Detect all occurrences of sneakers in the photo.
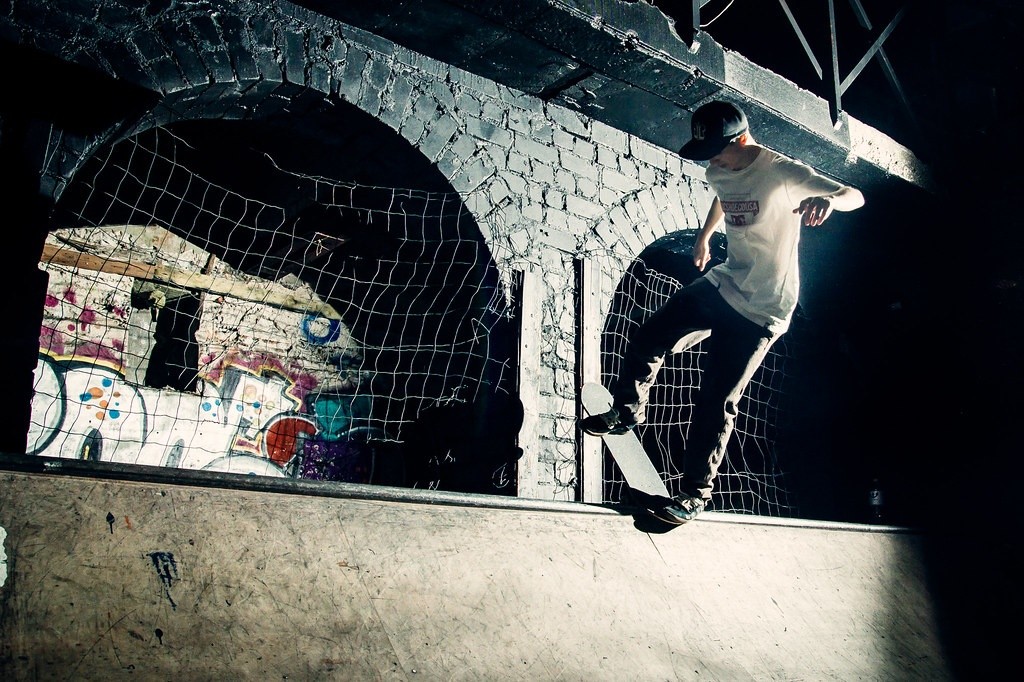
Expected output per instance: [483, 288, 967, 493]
[582, 407, 631, 435]
[663, 491, 705, 522]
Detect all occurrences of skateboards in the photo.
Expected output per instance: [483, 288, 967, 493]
[581, 383, 683, 525]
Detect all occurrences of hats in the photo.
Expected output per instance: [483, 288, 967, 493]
[678, 99, 750, 161]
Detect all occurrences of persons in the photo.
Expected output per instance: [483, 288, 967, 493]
[579, 98, 866, 527]
